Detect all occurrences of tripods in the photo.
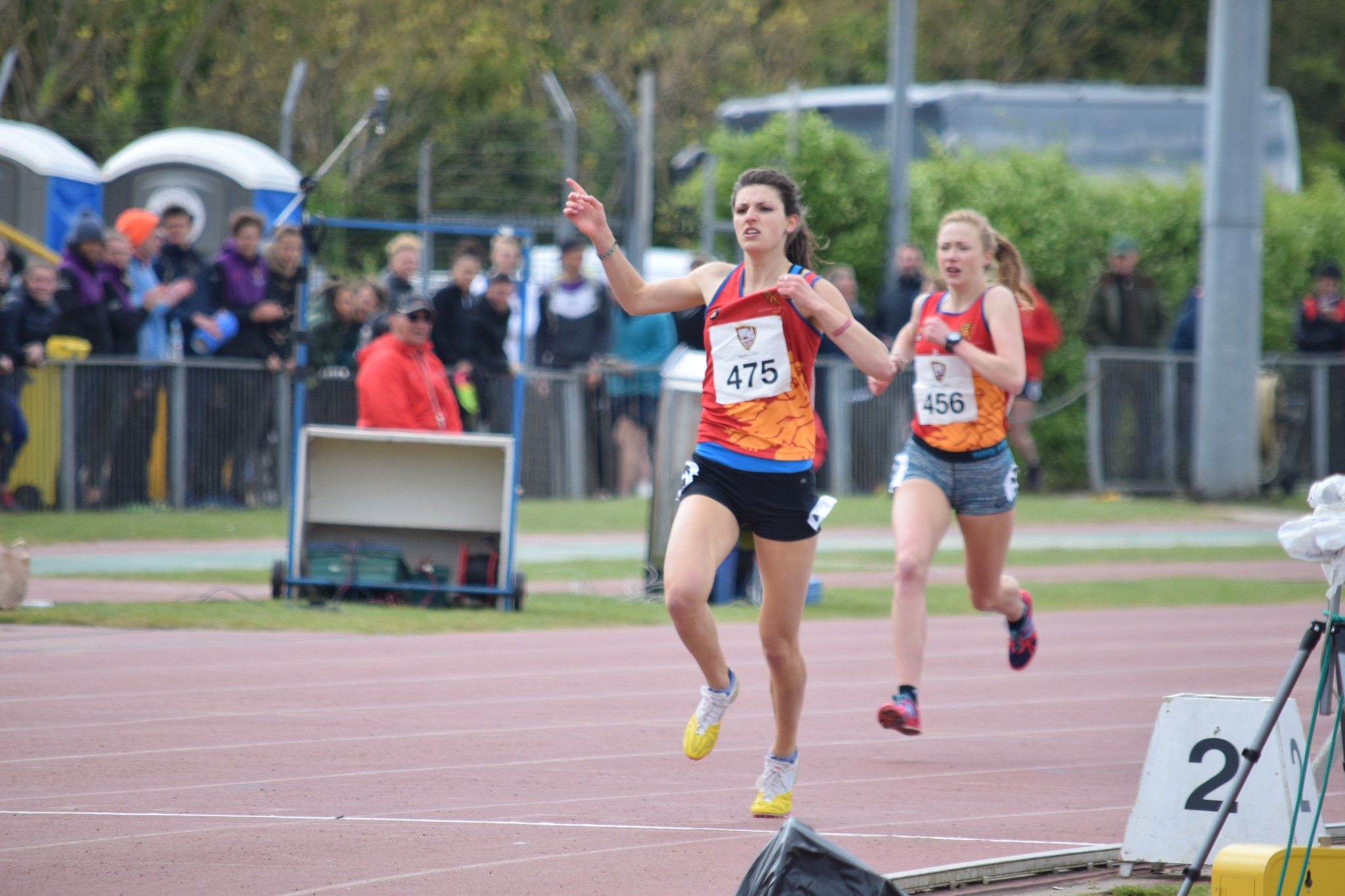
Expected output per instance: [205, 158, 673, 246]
[1178, 588, 1345, 896]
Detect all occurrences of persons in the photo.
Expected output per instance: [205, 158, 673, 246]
[869, 209, 1039, 738]
[1, 204, 1345, 522]
[564, 167, 895, 817]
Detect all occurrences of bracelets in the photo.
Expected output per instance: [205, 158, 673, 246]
[891, 355, 900, 374]
[897, 352, 906, 371]
[598, 240, 618, 260]
[829, 318, 853, 337]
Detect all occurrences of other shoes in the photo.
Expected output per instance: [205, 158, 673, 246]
[1026, 459, 1041, 489]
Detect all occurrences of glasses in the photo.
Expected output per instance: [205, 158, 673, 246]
[406, 311, 433, 322]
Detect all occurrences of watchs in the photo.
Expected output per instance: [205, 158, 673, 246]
[944, 332, 962, 352]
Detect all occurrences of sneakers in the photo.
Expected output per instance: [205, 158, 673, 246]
[681, 664, 739, 760]
[1005, 589, 1038, 671]
[878, 693, 922, 737]
[751, 750, 799, 817]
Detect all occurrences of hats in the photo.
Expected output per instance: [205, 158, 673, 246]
[1109, 238, 1137, 258]
[115, 208, 160, 246]
[73, 211, 106, 248]
[395, 292, 435, 315]
[1304, 259, 1341, 279]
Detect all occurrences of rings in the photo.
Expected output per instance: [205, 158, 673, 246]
[784, 274, 788, 281]
